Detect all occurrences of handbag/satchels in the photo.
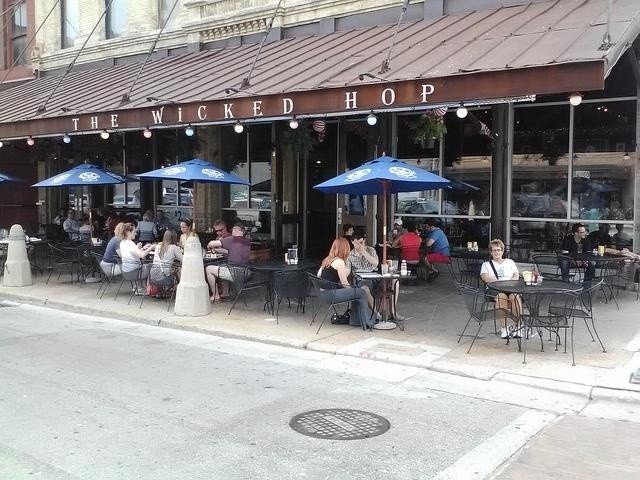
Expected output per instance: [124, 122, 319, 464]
[331, 308, 351, 324]
[164, 273, 179, 291]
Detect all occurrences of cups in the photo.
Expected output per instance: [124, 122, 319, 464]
[381, 264, 388, 275]
[536, 276, 543, 286]
[205, 254, 210, 258]
[598, 246, 605, 256]
[578, 244, 583, 253]
[467, 242, 479, 252]
[392, 260, 398, 269]
[523, 271, 532, 286]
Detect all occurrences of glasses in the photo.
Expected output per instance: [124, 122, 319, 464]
[492, 249, 501, 252]
[215, 229, 223, 232]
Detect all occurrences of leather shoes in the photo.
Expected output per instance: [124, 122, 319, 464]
[393, 313, 404, 320]
[434, 269, 440, 279]
[427, 273, 435, 283]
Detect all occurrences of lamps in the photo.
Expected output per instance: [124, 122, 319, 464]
[101, 128, 109, 140]
[144, 125, 152, 139]
[185, 123, 194, 137]
[568, 92, 584, 106]
[63, 131, 71, 143]
[26, 135, 34, 146]
[234, 119, 243, 134]
[367, 108, 377, 126]
[289, 115, 298, 130]
[457, 100, 468, 119]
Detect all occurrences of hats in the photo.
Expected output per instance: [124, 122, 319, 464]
[393, 219, 403, 226]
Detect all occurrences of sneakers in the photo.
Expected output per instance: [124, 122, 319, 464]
[221, 296, 230, 301]
[500, 326, 510, 338]
[513, 326, 523, 338]
[134, 288, 146, 295]
[211, 298, 221, 303]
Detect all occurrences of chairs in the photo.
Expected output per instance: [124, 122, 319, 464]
[0, 222, 318, 325]
[306, 269, 366, 335]
[375, 239, 640, 366]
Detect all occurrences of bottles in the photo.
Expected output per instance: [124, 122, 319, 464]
[533, 271, 540, 285]
[393, 230, 397, 241]
[401, 260, 407, 277]
[593, 249, 598, 256]
[93, 238, 103, 247]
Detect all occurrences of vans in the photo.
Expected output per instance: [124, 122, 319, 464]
[173, 187, 193, 207]
[112, 195, 138, 206]
[132, 187, 177, 205]
[454, 192, 564, 230]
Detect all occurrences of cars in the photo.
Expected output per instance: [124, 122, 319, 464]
[398, 197, 428, 213]
[397, 201, 470, 247]
[228, 192, 272, 209]
[558, 200, 580, 219]
[68, 193, 89, 208]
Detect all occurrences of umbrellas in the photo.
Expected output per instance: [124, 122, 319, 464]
[537, 175, 619, 219]
[313, 152, 453, 323]
[135, 157, 252, 238]
[252, 178, 271, 194]
[0, 172, 24, 183]
[419, 176, 481, 226]
[30, 160, 140, 278]
[178, 180, 193, 189]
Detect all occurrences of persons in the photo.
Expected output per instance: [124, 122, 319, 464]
[390, 219, 450, 282]
[587, 223, 632, 252]
[317, 238, 369, 327]
[480, 237, 522, 339]
[343, 223, 354, 250]
[150, 229, 183, 291]
[622, 247, 640, 291]
[179, 217, 199, 245]
[206, 220, 250, 304]
[101, 222, 156, 296]
[346, 231, 405, 321]
[135, 210, 168, 242]
[558, 222, 595, 292]
[55, 208, 121, 244]
